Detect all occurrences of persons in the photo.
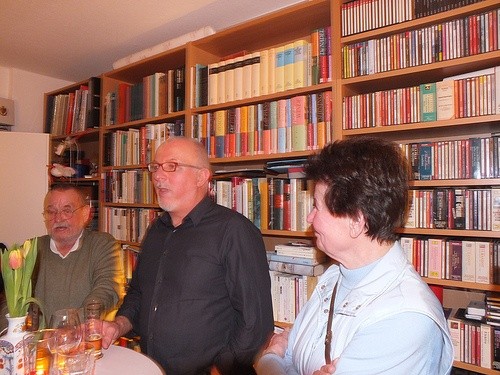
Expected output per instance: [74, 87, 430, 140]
[18, 183, 124, 329]
[254, 136, 454, 375]
[69, 136, 275, 375]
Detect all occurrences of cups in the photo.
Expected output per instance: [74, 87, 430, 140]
[53, 343, 95, 374]
[84, 299, 104, 360]
[48, 308, 83, 354]
[25, 305, 33, 331]
[24, 328, 58, 375]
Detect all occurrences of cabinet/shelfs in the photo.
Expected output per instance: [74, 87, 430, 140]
[41, 1, 500, 375]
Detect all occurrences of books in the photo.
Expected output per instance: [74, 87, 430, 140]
[209, 157, 317, 232]
[405, 185, 500, 231]
[117, 332, 143, 353]
[102, 66, 184, 126]
[189, 25, 333, 110]
[99, 168, 160, 205]
[396, 235, 500, 284]
[342, 64, 500, 181]
[103, 120, 183, 166]
[190, 91, 333, 159]
[119, 245, 141, 285]
[428, 285, 500, 370]
[340, 0, 500, 79]
[45, 76, 99, 136]
[262, 240, 342, 336]
[102, 207, 166, 243]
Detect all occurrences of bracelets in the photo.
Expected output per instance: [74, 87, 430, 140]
[257, 351, 276, 356]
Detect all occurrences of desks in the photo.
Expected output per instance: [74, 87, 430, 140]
[0, 332, 167, 375]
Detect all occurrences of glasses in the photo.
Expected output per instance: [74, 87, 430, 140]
[146, 162, 203, 172]
[42, 204, 88, 220]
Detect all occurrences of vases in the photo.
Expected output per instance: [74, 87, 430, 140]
[4, 313, 28, 345]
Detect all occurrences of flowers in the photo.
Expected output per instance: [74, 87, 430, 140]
[0, 236, 45, 351]
